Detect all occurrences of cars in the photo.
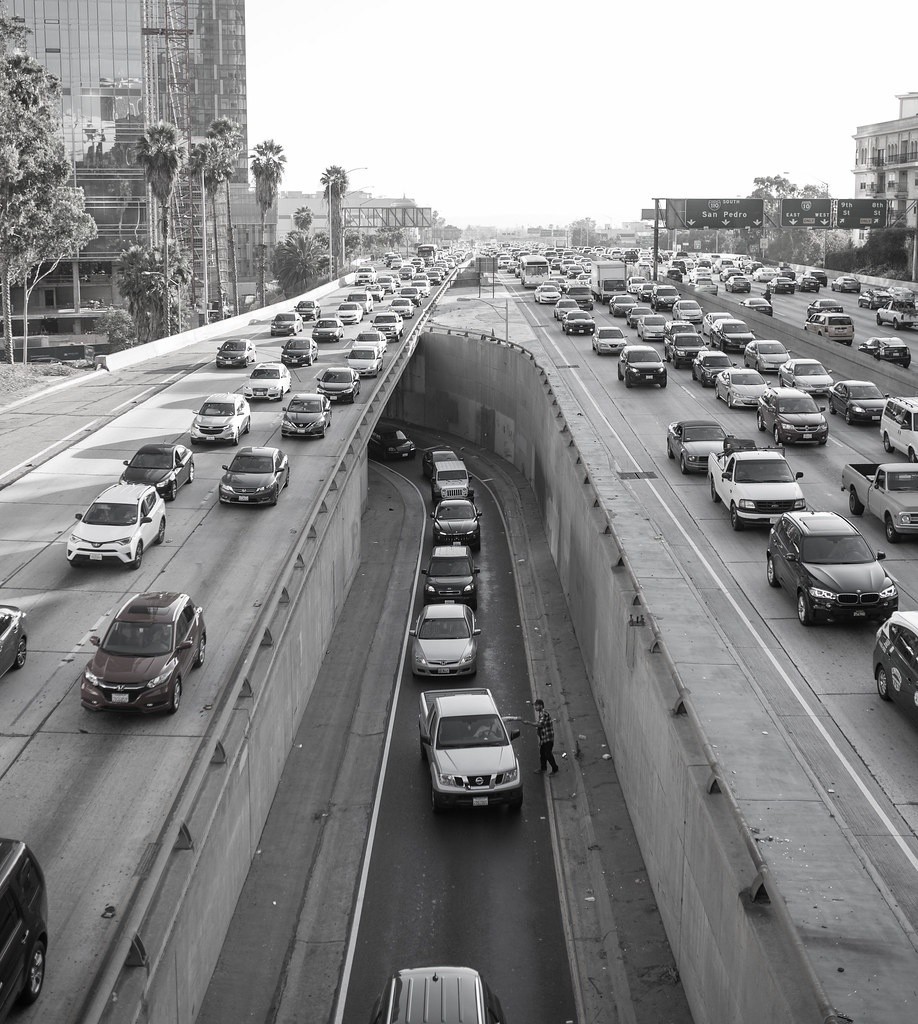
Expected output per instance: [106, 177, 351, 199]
[316, 368, 361, 405]
[280, 394, 332, 439]
[1, 837, 50, 1024]
[371, 965, 507, 1024]
[218, 446, 289, 507]
[190, 394, 251, 446]
[79, 589, 207, 720]
[408, 604, 481, 680]
[119, 444, 195, 503]
[484, 237, 918, 723]
[367, 424, 482, 613]
[243, 362, 292, 402]
[214, 246, 466, 379]
[0, 604, 29, 677]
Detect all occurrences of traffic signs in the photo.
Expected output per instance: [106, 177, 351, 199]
[684, 198, 889, 229]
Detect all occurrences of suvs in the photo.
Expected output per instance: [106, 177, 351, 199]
[65, 483, 166, 571]
[757, 387, 829, 446]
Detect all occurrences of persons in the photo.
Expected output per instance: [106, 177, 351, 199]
[523, 699, 559, 775]
[161, 455, 172, 468]
[149, 627, 171, 651]
[841, 538, 869, 559]
[259, 462, 272, 470]
[800, 400, 816, 413]
[473, 716, 522, 738]
[125, 508, 144, 520]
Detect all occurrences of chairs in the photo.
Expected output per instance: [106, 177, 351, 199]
[119, 625, 140, 639]
[141, 455, 156, 466]
[836, 538, 867, 558]
[148, 623, 168, 641]
[447, 721, 466, 740]
[470, 717, 493, 737]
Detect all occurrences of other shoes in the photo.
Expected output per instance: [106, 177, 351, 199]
[535, 768, 545, 773]
[549, 772, 555, 776]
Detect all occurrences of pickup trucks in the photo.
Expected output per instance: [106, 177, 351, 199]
[839, 461, 918, 545]
[706, 447, 805, 531]
[418, 687, 524, 816]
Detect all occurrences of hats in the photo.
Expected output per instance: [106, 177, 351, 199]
[534, 699, 544, 706]
